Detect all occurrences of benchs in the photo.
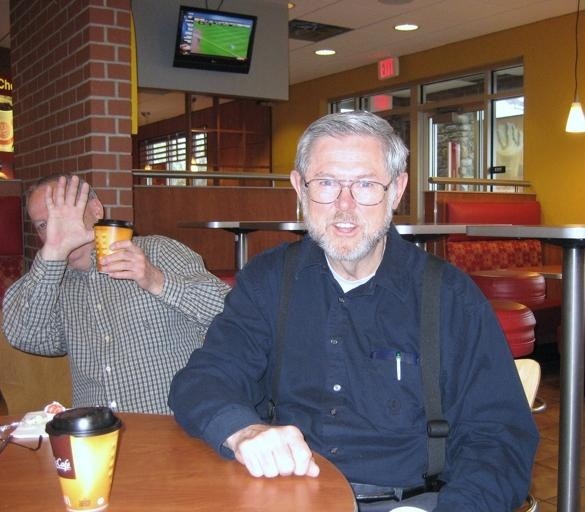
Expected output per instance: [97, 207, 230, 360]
[424, 192, 561, 345]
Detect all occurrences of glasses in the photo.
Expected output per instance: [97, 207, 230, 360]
[304, 179, 392, 206]
[0, 435, 42, 454]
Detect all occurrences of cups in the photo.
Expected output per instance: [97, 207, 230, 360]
[45, 405, 122, 512]
[92, 219, 134, 276]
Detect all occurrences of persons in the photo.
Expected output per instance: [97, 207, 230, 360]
[1, 171, 231, 416]
[163, 108, 537, 512]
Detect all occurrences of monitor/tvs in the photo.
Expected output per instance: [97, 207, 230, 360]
[173, 5, 257, 74]
